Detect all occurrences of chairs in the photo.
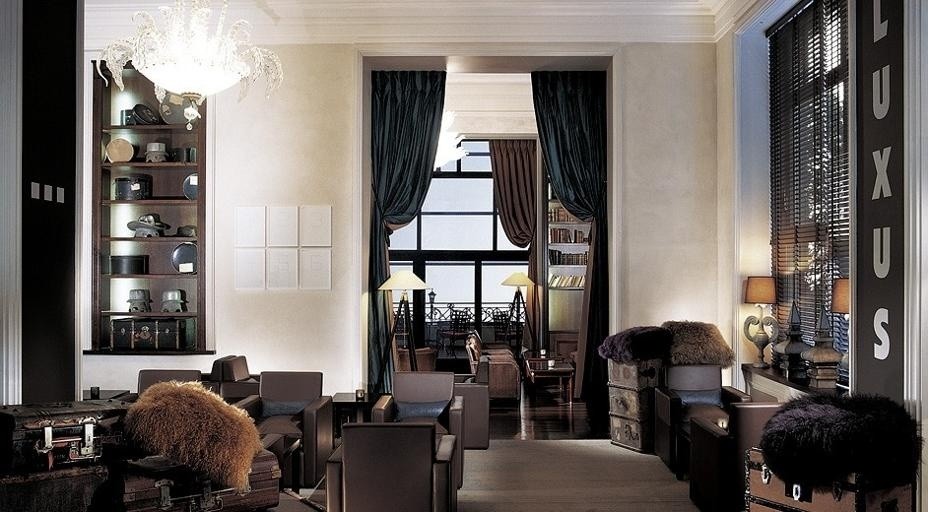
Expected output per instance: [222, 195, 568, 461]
[118, 370, 200, 404]
[200, 354, 236, 395]
[652, 362, 787, 512]
[325, 421, 457, 511]
[220, 356, 260, 404]
[465, 330, 519, 412]
[397, 348, 491, 450]
[435, 304, 523, 357]
[231, 371, 333, 492]
[371, 372, 464, 490]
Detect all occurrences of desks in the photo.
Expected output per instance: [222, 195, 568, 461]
[83, 390, 130, 400]
[331, 392, 378, 439]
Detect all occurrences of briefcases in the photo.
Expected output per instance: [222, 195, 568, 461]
[117, 448, 281, 511]
[744, 446, 917, 512]
[1, 399, 135, 470]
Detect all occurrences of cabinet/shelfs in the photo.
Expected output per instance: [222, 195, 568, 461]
[606, 357, 661, 454]
[91, 58, 208, 357]
[541, 175, 593, 358]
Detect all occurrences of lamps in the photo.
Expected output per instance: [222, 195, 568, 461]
[376, 270, 433, 395]
[829, 277, 850, 398]
[500, 272, 535, 360]
[92, 0, 286, 122]
[743, 274, 780, 368]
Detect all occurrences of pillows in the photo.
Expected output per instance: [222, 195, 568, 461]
[757, 390, 923, 495]
[597, 319, 737, 370]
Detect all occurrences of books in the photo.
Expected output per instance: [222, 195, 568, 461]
[548, 180, 594, 288]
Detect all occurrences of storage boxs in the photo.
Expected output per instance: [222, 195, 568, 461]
[101, 441, 281, 511]
